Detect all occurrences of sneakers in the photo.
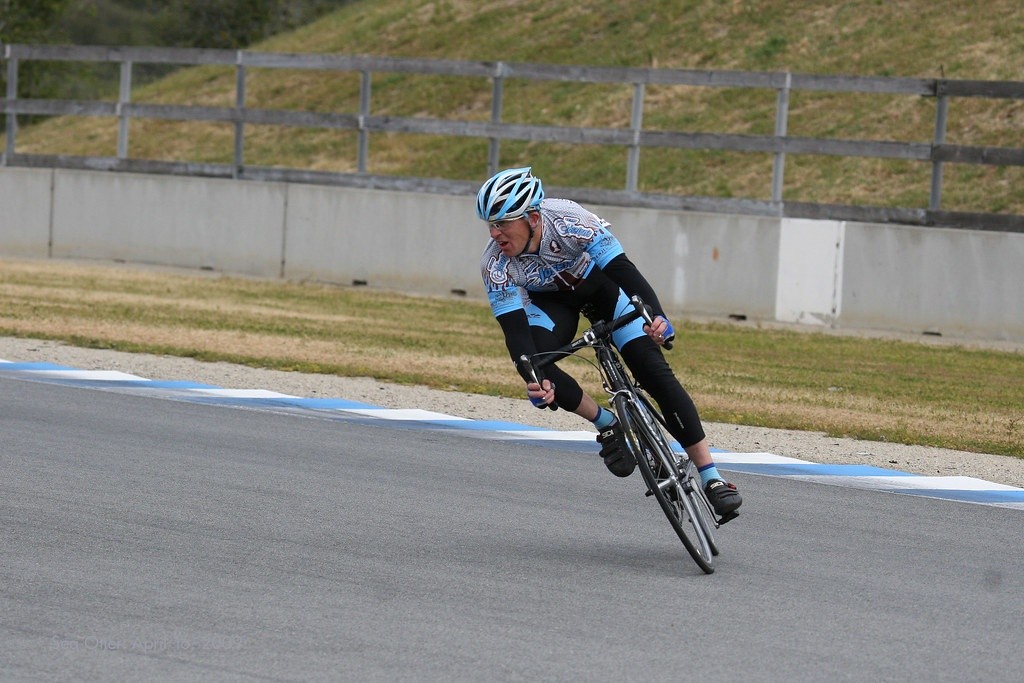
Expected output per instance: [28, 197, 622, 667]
[597, 407, 636, 477]
[705, 480, 742, 514]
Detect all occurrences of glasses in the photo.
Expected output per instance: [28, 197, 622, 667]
[488, 220, 513, 231]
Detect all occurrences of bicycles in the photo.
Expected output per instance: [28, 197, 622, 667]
[516, 292, 740, 574]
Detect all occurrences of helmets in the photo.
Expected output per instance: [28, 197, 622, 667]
[475, 167, 544, 221]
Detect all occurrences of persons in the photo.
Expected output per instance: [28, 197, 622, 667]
[476, 167, 742, 516]
